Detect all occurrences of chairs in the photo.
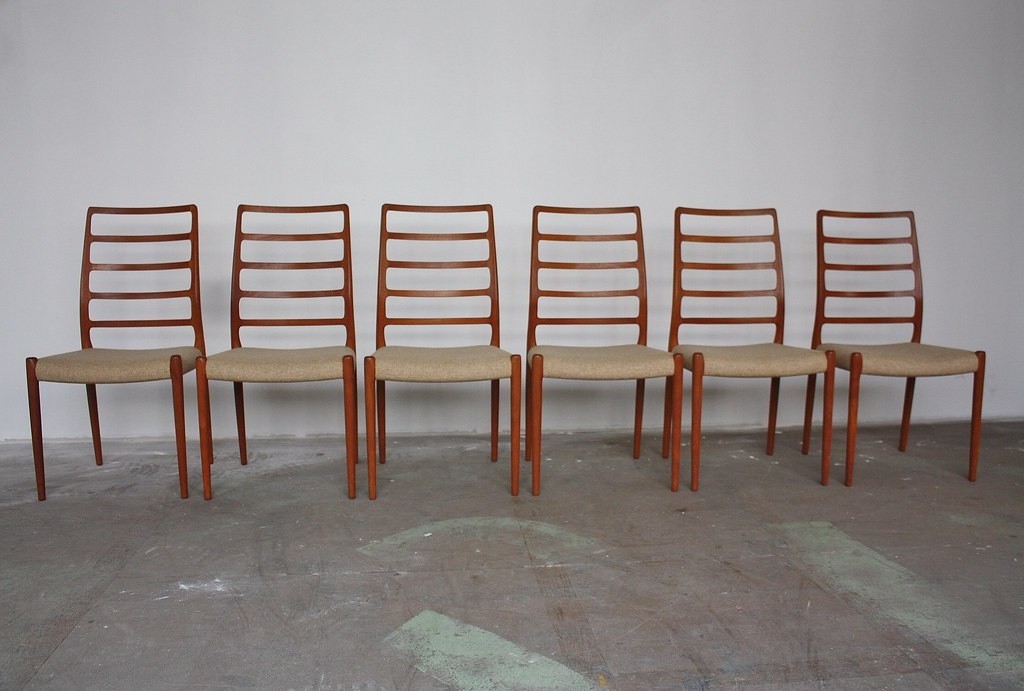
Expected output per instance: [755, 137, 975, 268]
[195, 203, 358, 500]
[662, 207, 836, 492]
[25, 204, 213, 501]
[525, 206, 685, 496]
[801, 209, 987, 487]
[363, 203, 521, 500]
